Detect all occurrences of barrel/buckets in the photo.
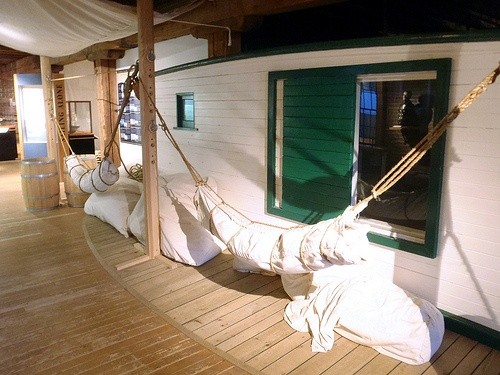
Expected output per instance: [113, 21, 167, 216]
[62, 153, 97, 209]
[21, 158, 59, 211]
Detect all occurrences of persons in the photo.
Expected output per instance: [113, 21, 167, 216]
[398, 90, 415, 126]
